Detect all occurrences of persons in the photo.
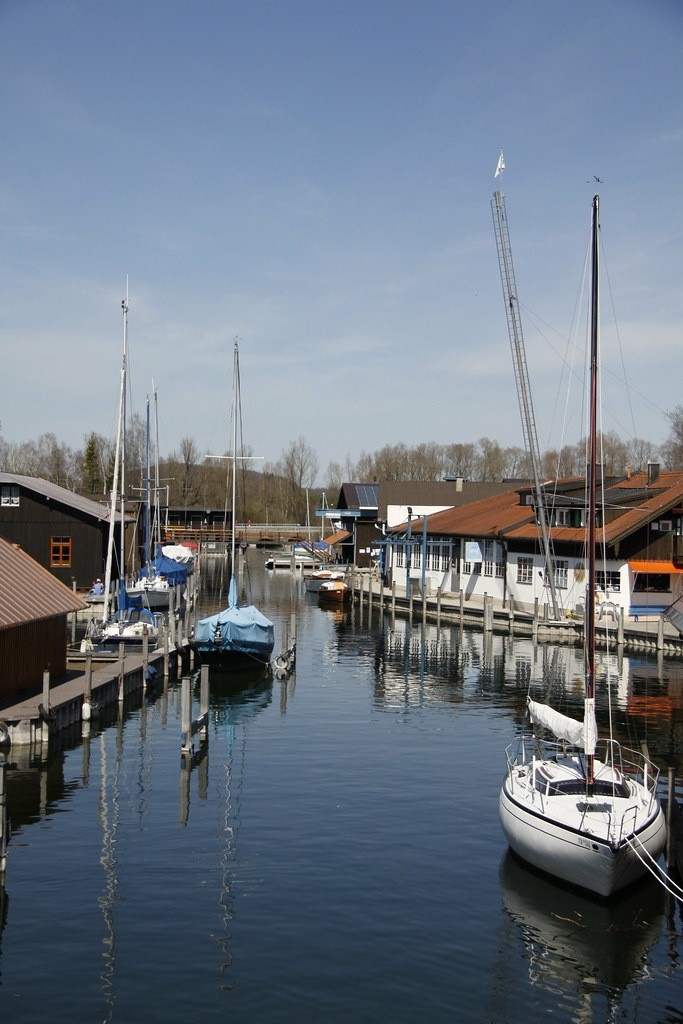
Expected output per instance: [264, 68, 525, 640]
[92, 578, 104, 595]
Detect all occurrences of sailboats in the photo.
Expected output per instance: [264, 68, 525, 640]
[499, 166, 672, 901]
[265, 489, 364, 601]
[190, 335, 275, 671]
[94, 272, 197, 640]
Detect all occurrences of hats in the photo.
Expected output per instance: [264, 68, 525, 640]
[95, 579, 102, 582]
